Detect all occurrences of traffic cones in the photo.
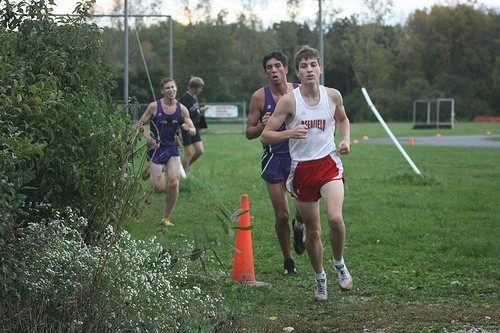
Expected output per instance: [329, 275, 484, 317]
[409, 137, 414, 145]
[230, 193, 257, 284]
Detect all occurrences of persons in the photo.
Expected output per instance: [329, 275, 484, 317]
[180, 77, 208, 170]
[259, 46, 354, 303]
[245, 50, 307, 276]
[136, 77, 197, 227]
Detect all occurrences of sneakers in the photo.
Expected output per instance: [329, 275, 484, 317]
[314, 273, 328, 301]
[333, 262, 352, 290]
[283, 257, 296, 275]
[142, 166, 151, 180]
[159, 218, 175, 226]
[292, 219, 306, 255]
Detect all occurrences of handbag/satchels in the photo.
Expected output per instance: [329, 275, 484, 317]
[200, 104, 208, 129]
